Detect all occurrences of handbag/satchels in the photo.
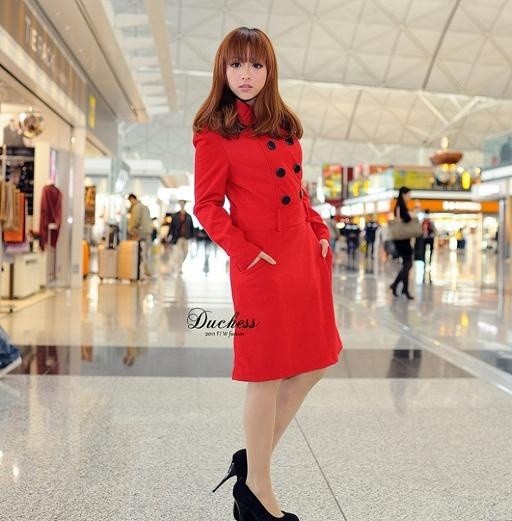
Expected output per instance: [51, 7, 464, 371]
[382, 204, 423, 241]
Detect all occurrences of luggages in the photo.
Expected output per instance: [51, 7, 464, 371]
[116, 239, 146, 281]
[97, 244, 117, 280]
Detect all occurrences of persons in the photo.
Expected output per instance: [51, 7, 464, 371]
[190, 25, 345, 521]
[0, 327, 22, 381]
[125, 194, 230, 279]
[385, 331, 422, 409]
[325, 187, 468, 300]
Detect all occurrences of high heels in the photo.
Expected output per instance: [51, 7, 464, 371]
[402, 288, 415, 299]
[232, 478, 300, 521]
[389, 283, 399, 296]
[213, 448, 247, 521]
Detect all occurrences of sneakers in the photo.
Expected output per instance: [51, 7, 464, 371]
[0, 347, 22, 379]
